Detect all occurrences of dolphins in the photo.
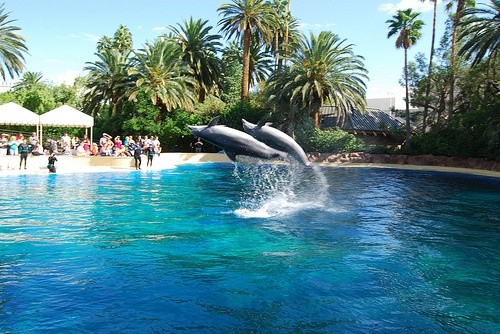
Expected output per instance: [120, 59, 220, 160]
[187, 115, 288, 163]
[241, 118, 312, 167]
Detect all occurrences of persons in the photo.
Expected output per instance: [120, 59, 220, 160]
[195, 141, 203, 153]
[47, 153, 57, 173]
[19, 139, 28, 170]
[0, 133, 164, 156]
[132, 140, 141, 169]
[145, 142, 155, 168]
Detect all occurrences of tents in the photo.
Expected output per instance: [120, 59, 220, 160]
[0, 102, 39, 139]
[40, 105, 94, 153]
